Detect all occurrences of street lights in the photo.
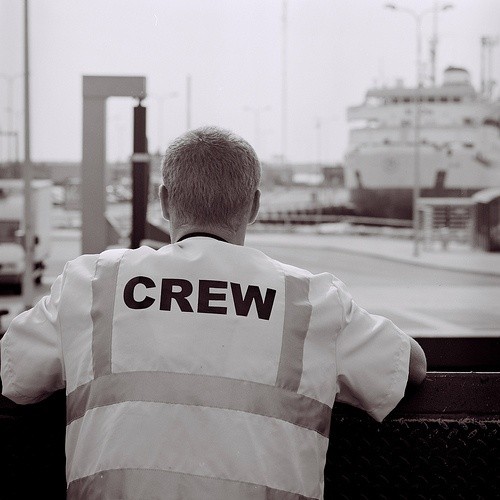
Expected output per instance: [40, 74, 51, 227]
[385, 1, 455, 257]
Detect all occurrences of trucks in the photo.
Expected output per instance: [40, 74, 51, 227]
[1, 178, 49, 294]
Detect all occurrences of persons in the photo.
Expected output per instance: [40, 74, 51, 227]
[0, 127, 426, 500]
